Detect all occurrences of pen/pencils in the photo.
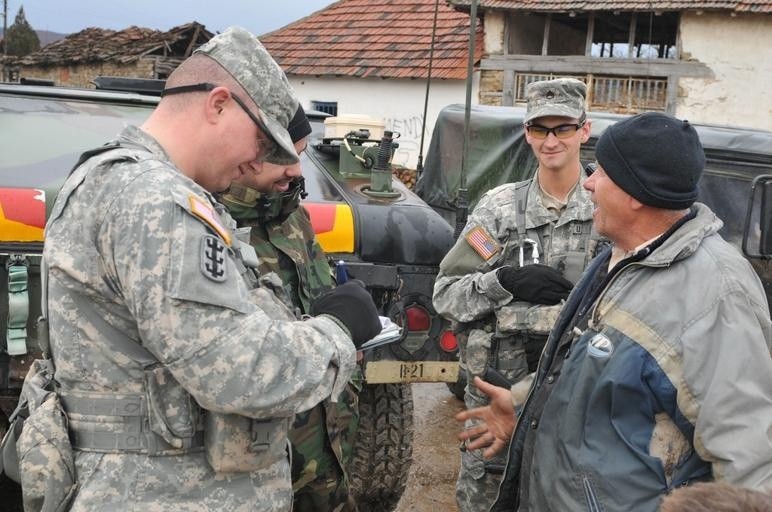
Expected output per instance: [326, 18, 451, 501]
[336, 259, 349, 283]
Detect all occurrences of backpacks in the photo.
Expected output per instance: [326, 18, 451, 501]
[14, 356, 78, 511]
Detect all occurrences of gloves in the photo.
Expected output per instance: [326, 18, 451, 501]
[525, 339, 546, 373]
[497, 263, 574, 305]
[309, 279, 382, 347]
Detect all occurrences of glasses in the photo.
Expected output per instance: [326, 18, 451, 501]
[204, 81, 278, 162]
[524, 119, 585, 140]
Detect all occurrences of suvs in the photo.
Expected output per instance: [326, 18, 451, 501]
[1, 2, 477, 512]
[410, 1, 771, 406]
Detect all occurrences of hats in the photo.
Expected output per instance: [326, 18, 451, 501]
[192, 25, 312, 165]
[524, 78, 588, 124]
[594, 112, 705, 210]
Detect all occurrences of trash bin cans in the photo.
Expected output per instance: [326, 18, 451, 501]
[323, 114, 385, 146]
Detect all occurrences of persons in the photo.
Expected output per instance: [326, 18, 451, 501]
[43, 24, 384, 510]
[449, 109, 771, 511]
[218, 98, 370, 511]
[654, 476, 770, 511]
[428, 76, 610, 510]
